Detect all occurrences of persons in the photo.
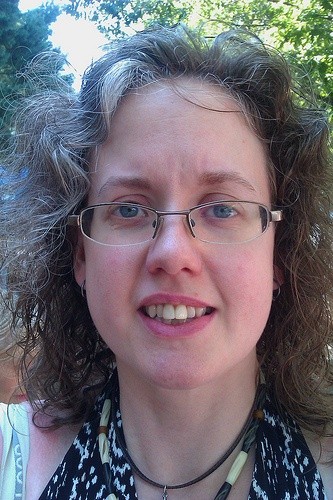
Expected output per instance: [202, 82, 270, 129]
[0, 21, 332, 500]
[0, 288, 46, 405]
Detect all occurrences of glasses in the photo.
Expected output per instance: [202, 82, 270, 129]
[76, 201, 282, 245]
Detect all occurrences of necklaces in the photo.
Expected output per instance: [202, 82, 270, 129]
[99, 366, 266, 500]
